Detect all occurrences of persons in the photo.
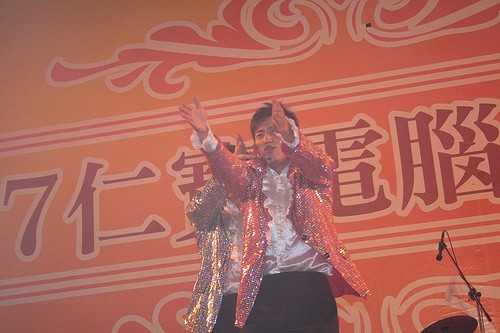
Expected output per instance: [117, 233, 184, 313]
[181, 134, 247, 333]
[179, 94, 372, 333]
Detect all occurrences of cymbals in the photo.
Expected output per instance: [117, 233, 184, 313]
[420, 315, 477, 333]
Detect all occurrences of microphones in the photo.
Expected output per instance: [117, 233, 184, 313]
[262, 157, 271, 160]
[436, 232, 445, 261]
